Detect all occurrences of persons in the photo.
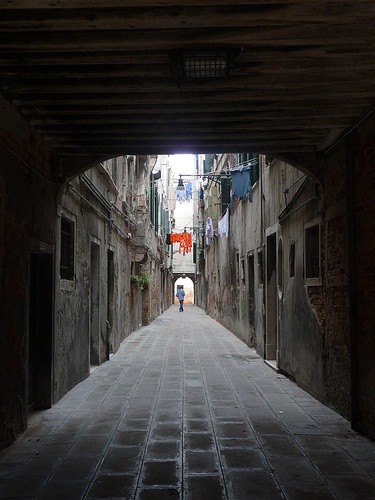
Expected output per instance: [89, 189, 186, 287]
[176, 285, 185, 312]
